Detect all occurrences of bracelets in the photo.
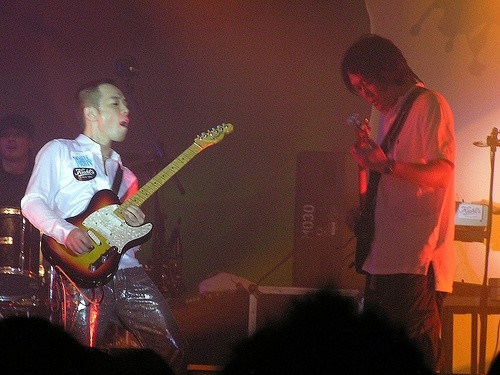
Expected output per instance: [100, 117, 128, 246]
[381, 158, 395, 174]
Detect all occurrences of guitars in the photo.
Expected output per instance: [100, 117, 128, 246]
[41, 122, 234, 289]
[347, 112, 380, 275]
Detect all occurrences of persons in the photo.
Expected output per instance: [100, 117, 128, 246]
[340, 35, 455, 374]
[0, 110, 37, 207]
[20, 78, 188, 374]
[218, 285, 439, 373]
[0, 313, 125, 374]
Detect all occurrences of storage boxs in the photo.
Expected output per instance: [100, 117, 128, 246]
[248, 288, 358, 339]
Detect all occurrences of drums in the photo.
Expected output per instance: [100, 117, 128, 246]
[0, 206, 41, 302]
[0, 240, 65, 330]
